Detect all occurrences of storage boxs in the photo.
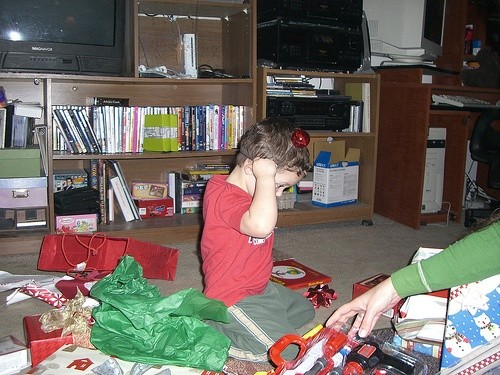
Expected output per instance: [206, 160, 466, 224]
[0, 334, 32, 375]
[13, 102, 42, 119]
[134, 196, 174, 219]
[52, 169, 88, 193]
[312, 148, 360, 208]
[132, 182, 168, 200]
[351, 273, 405, 317]
[54, 187, 102, 225]
[23, 315, 93, 368]
[0, 176, 48, 209]
[55, 213, 98, 234]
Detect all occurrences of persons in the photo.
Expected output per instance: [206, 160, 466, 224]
[199, 117, 317, 361]
[325, 221, 498, 340]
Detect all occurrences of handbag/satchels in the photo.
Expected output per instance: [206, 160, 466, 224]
[37, 235, 180, 281]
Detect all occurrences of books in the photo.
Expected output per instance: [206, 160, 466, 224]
[266, 74, 318, 97]
[0, 98, 251, 225]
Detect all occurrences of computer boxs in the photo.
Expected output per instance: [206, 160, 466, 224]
[422, 128, 447, 215]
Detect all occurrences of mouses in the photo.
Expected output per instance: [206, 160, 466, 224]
[496, 100, 500, 107]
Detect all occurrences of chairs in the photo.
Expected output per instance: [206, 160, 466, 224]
[465, 110, 500, 228]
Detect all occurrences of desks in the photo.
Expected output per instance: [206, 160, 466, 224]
[371, 64, 500, 231]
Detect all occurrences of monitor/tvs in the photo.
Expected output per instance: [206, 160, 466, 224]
[421, 0, 447, 61]
[0, 0, 135, 78]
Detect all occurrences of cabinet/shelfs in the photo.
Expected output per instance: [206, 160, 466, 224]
[0, 0, 381, 257]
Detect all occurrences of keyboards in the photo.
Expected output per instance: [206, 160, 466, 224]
[432, 93, 496, 108]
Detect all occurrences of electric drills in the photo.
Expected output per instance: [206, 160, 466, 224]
[343, 341, 414, 375]
